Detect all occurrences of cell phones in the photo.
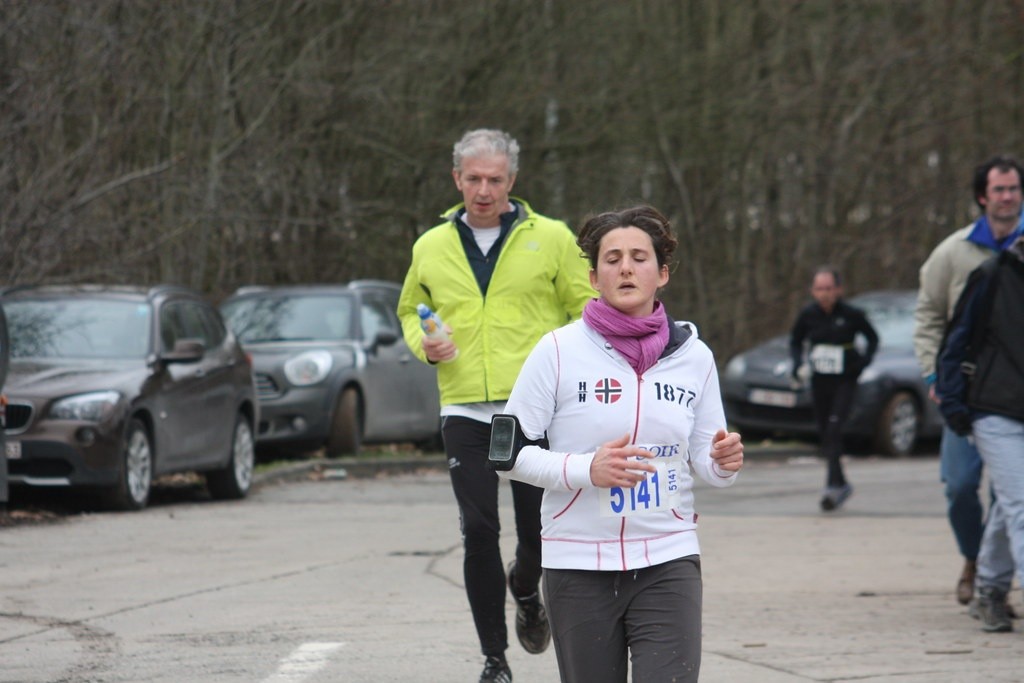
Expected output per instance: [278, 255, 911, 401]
[487, 414, 521, 471]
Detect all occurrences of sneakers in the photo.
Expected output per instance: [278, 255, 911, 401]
[967, 585, 1012, 632]
[479, 658, 513, 683]
[821, 482, 854, 512]
[507, 560, 552, 654]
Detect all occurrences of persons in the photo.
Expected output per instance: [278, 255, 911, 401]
[397, 129, 600, 683]
[487, 206, 744, 683]
[789, 269, 879, 511]
[913, 155, 1024, 632]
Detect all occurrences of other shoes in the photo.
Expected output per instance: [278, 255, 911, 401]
[1006, 602, 1023, 619]
[956, 563, 976, 604]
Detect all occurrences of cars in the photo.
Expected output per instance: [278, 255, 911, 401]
[719, 291, 945, 457]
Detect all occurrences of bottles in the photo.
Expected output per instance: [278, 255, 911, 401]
[417, 303, 459, 363]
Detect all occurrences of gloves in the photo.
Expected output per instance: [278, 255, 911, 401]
[792, 358, 806, 382]
[939, 399, 973, 437]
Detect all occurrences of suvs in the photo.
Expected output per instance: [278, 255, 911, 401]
[0, 282, 261, 509]
[212, 279, 445, 461]
